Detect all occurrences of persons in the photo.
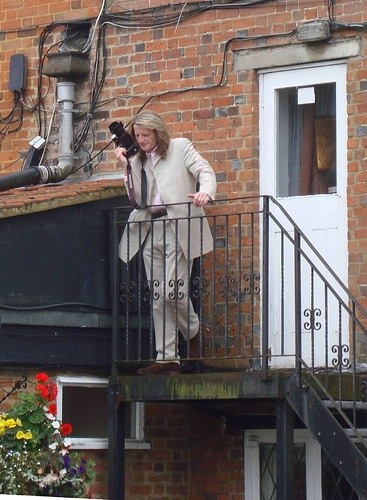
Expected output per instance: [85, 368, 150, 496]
[114, 110, 218, 375]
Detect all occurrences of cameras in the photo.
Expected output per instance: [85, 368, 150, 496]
[109, 121, 139, 159]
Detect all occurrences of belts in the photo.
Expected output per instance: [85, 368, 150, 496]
[151, 207, 168, 220]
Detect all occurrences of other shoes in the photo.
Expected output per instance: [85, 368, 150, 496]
[137, 361, 180, 375]
[179, 334, 203, 372]
